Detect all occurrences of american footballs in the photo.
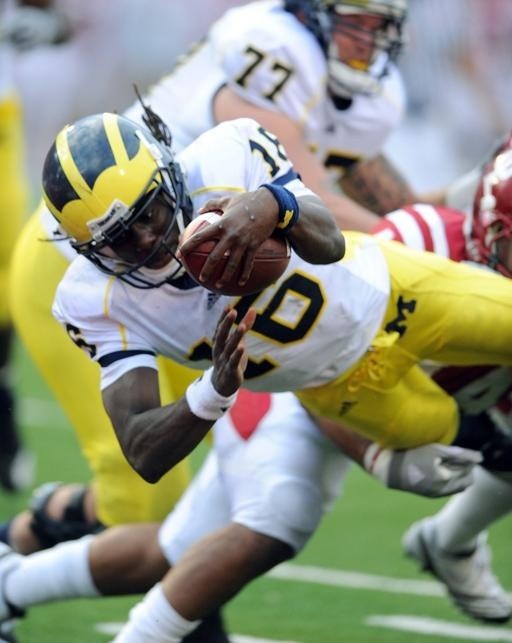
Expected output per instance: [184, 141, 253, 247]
[178, 211, 292, 294]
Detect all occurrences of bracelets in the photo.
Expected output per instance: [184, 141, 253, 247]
[185, 365, 239, 422]
[258, 183, 300, 235]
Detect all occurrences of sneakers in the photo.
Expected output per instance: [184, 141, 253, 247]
[401, 517, 512, 622]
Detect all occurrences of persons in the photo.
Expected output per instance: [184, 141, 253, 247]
[41, 112, 512, 483]
[0, 129, 512, 643]
[2, 1, 75, 497]
[1, 0, 446, 643]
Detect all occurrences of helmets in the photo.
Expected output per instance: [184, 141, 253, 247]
[42, 113, 193, 288]
[285, 0, 409, 99]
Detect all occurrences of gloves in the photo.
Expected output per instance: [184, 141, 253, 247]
[364, 443, 484, 498]
